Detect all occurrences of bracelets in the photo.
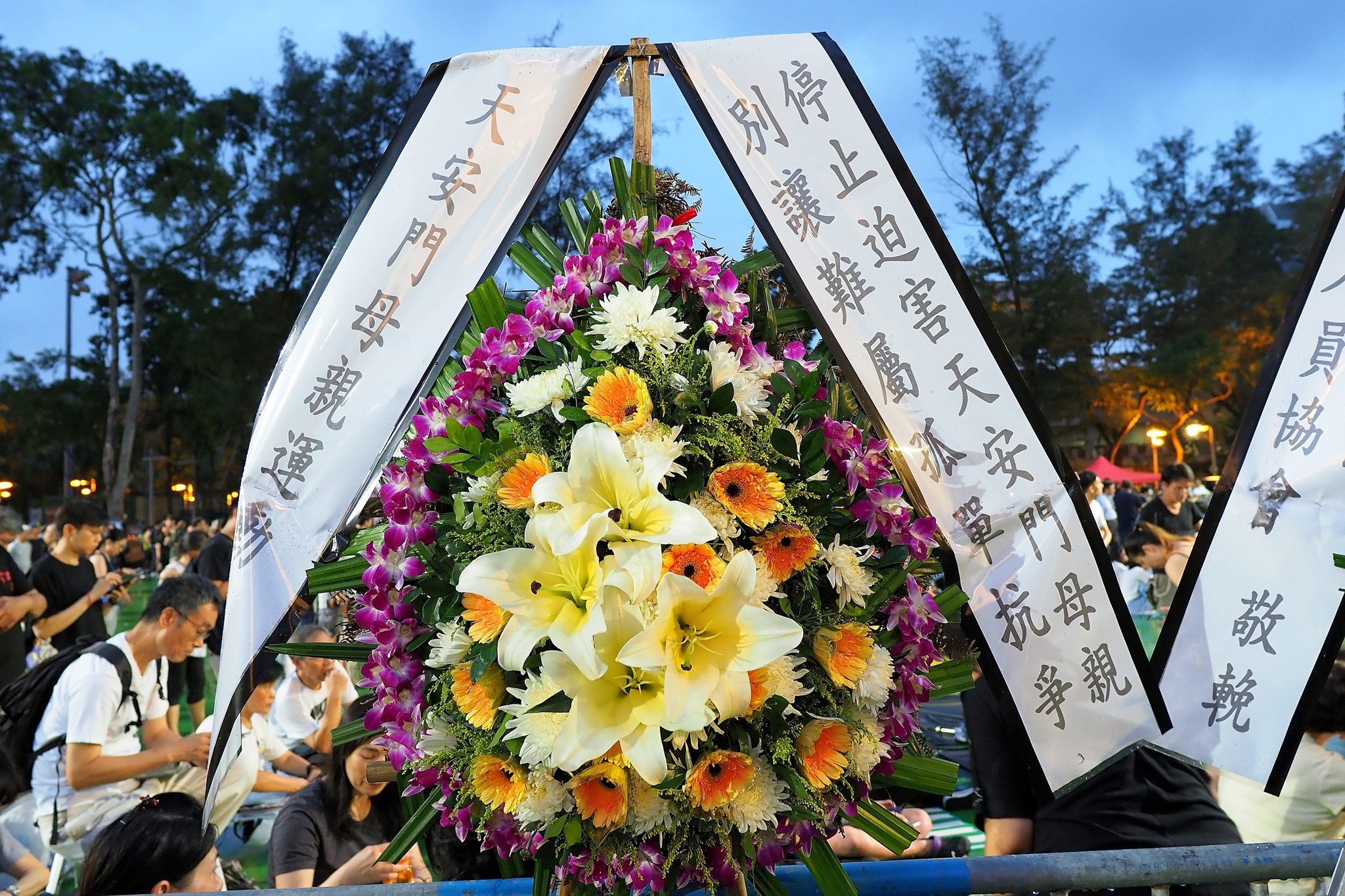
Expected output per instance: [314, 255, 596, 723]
[87, 592, 93, 605]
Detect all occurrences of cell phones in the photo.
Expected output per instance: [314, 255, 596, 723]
[106, 575, 134, 594]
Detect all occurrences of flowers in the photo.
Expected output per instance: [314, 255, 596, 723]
[265, 153, 984, 895]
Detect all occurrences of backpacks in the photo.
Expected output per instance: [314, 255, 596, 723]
[0, 632, 162, 805]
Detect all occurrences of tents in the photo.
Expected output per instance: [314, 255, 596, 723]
[1085, 455, 1164, 487]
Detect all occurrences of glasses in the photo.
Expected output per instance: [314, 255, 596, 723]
[174, 607, 211, 641]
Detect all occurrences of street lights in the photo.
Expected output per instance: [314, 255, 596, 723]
[1146, 427, 1169, 486]
[169, 483, 195, 522]
[61, 266, 92, 504]
[1186, 424, 1219, 490]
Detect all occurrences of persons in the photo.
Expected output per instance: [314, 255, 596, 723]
[1077, 461, 1219, 628]
[802, 800, 973, 859]
[1219, 665, 1345, 896]
[0, 496, 434, 896]
[937, 547, 1250, 896]
